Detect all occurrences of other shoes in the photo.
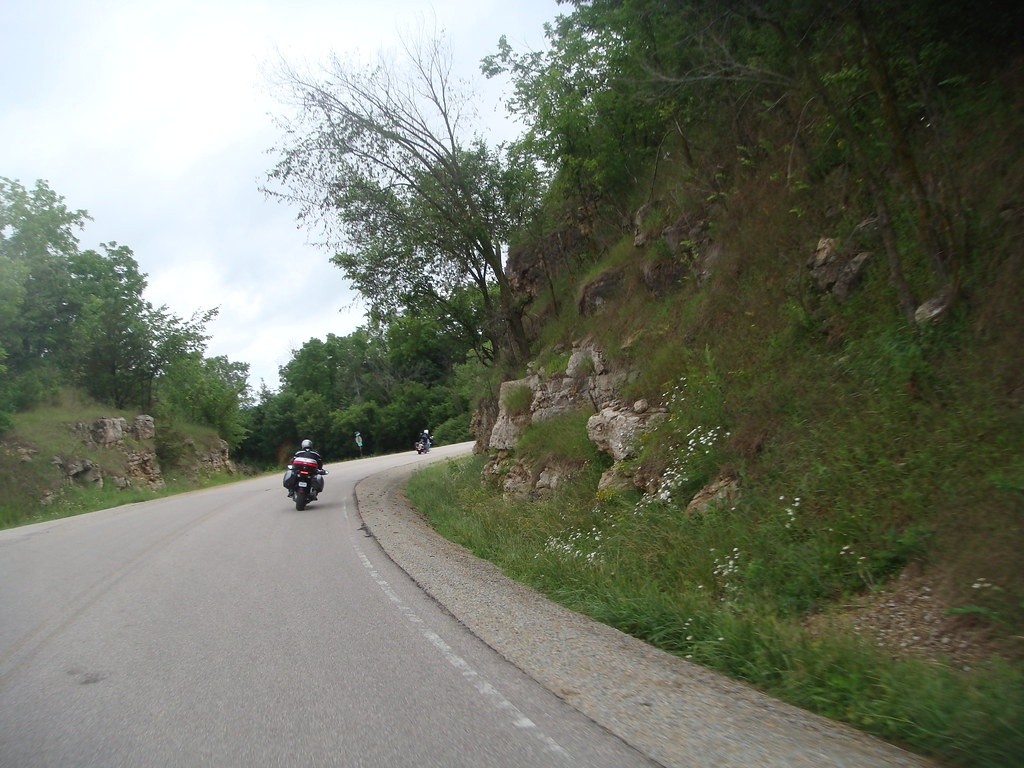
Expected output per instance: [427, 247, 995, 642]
[311, 495, 317, 500]
[287, 491, 294, 497]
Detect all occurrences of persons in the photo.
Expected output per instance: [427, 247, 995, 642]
[420, 430, 430, 452]
[285, 439, 323, 501]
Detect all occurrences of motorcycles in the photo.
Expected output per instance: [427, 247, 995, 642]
[415, 433, 434, 454]
[288, 457, 329, 511]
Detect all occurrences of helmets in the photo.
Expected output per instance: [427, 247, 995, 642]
[301, 439, 313, 449]
[424, 429, 429, 434]
